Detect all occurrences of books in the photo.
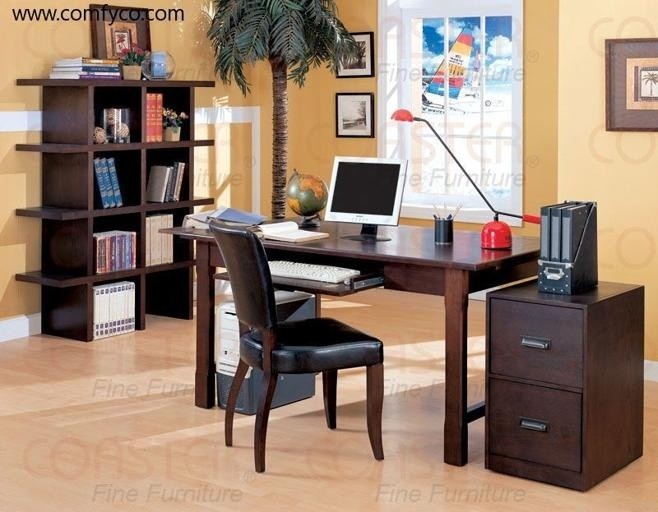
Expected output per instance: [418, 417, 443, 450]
[258, 221, 330, 243]
[49, 57, 121, 81]
[93, 92, 186, 275]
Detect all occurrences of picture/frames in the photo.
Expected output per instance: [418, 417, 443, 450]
[603, 37, 657, 131]
[334, 30, 375, 78]
[90, 2, 153, 61]
[333, 92, 374, 137]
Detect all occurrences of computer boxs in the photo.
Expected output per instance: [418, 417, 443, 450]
[215, 290, 316, 414]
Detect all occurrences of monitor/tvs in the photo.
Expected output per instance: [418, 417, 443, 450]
[325, 154, 408, 243]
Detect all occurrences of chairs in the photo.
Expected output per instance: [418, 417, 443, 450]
[205, 217, 388, 474]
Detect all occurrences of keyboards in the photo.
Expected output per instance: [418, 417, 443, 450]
[268, 259, 362, 284]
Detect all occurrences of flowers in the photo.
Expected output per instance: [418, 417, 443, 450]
[159, 105, 189, 133]
[116, 42, 147, 66]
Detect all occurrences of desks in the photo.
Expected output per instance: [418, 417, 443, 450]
[158, 211, 540, 468]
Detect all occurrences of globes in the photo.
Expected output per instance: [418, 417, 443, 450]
[286, 168, 328, 228]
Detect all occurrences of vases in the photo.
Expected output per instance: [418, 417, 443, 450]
[121, 65, 141, 80]
[162, 126, 180, 141]
[102, 106, 130, 144]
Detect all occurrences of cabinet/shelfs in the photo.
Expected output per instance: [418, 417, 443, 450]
[16, 75, 217, 342]
[485, 277, 643, 495]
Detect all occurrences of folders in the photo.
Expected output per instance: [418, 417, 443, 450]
[206, 206, 267, 226]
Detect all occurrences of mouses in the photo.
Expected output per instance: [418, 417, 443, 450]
[344, 278, 350, 286]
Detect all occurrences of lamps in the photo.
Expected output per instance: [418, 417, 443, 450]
[389, 109, 540, 250]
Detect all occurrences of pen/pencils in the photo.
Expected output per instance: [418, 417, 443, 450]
[432, 200, 462, 220]
